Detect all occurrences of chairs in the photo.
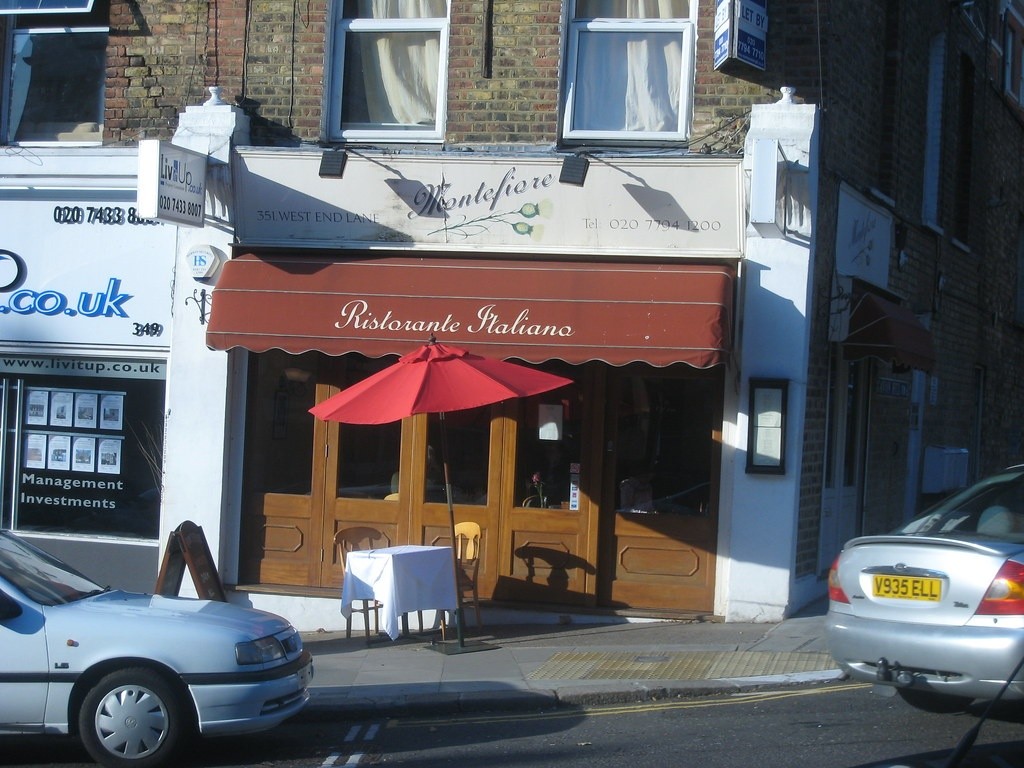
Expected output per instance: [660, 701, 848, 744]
[618, 479, 653, 512]
[332, 527, 392, 642]
[524, 495, 556, 509]
[431, 521, 482, 643]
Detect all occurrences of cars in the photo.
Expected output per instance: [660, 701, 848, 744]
[0, 530, 313, 768]
[824, 463, 1024, 714]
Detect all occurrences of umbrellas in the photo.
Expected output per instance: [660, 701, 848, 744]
[307, 332, 573, 645]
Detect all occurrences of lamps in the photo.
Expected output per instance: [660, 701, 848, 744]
[558, 151, 591, 186]
[317, 146, 348, 178]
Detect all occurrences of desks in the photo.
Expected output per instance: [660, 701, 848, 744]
[337, 544, 456, 643]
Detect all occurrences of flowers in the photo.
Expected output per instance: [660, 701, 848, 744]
[531, 472, 544, 506]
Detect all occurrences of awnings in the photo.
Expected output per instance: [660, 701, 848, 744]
[206, 254, 735, 369]
[841, 290, 935, 376]
